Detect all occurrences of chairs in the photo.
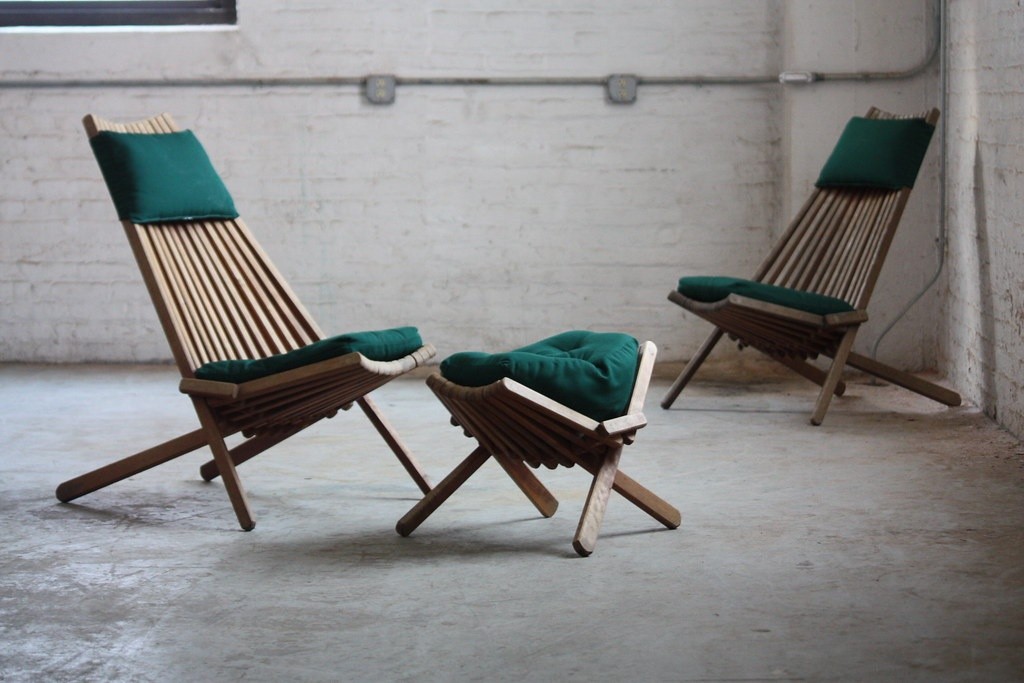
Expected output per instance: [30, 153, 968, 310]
[661, 106, 962, 428]
[55, 110, 438, 530]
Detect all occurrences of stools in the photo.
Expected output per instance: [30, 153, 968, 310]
[393, 329, 682, 556]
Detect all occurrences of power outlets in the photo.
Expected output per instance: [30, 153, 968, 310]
[608, 73, 637, 105]
[365, 73, 396, 105]
